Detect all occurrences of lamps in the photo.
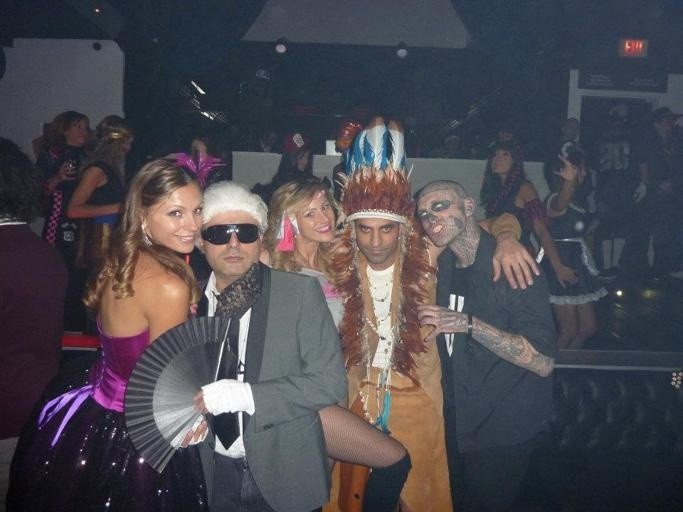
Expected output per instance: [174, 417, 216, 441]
[275, 36, 288, 55]
[396, 39, 408, 59]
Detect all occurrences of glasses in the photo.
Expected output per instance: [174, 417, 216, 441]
[200, 223, 260, 245]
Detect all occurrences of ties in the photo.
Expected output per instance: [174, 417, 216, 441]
[210, 305, 253, 450]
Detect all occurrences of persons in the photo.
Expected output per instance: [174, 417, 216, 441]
[479, 107, 682, 350]
[2, 110, 558, 510]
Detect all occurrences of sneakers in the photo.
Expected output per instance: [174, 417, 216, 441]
[603, 266, 617, 279]
